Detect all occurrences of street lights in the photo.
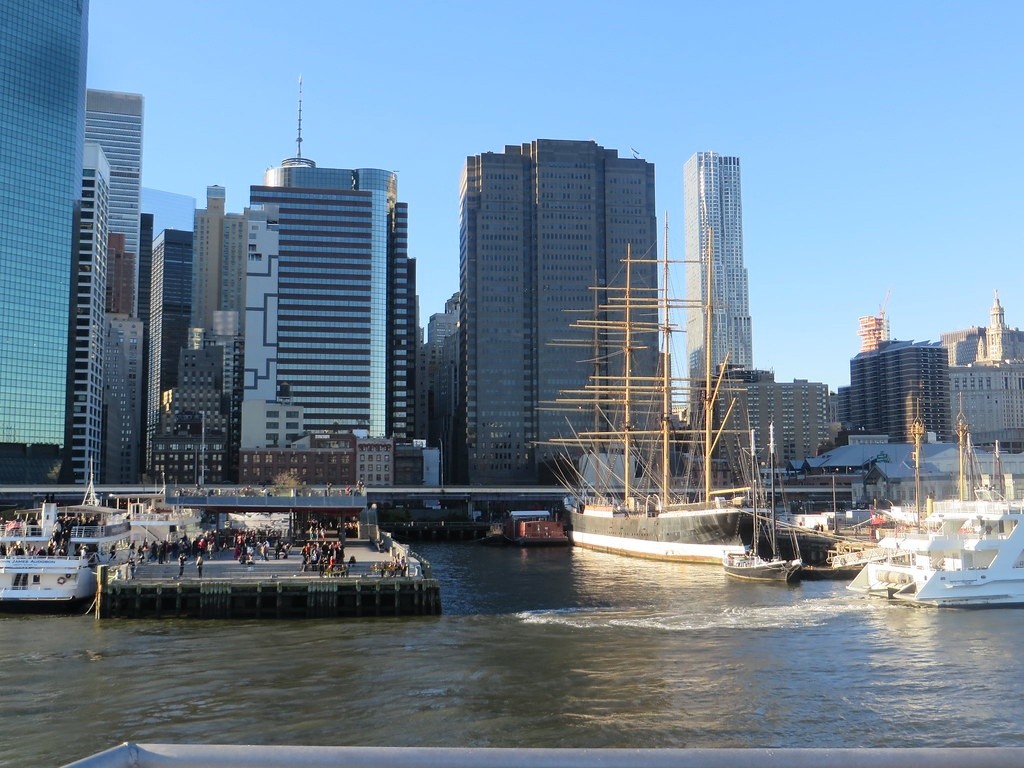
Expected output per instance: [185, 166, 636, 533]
[198, 410, 206, 486]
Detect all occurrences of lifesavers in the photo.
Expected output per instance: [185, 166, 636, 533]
[58, 576, 66, 585]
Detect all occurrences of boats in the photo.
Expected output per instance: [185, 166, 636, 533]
[723, 423, 805, 586]
[826, 391, 1024, 608]
[484, 509, 570, 544]
[0, 456, 136, 605]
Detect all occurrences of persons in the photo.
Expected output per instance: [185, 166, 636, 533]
[2, 513, 103, 562]
[108, 516, 346, 579]
[178, 481, 366, 497]
[984, 522, 993, 535]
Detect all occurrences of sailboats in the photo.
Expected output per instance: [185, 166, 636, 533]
[529, 210, 774, 565]
